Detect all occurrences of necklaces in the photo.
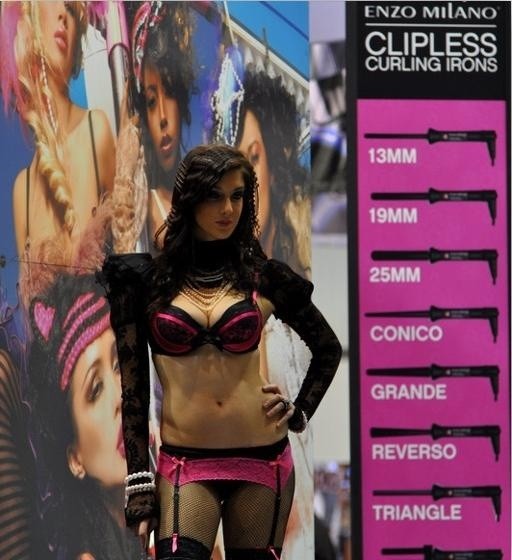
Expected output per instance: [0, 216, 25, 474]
[177, 265, 237, 313]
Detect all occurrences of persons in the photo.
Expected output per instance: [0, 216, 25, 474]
[109, 2, 203, 255]
[24, 273, 165, 559]
[1, 1, 116, 309]
[203, 58, 312, 282]
[101, 143, 342, 560]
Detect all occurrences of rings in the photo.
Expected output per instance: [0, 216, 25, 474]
[281, 398, 290, 413]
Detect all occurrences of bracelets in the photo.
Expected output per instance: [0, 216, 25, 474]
[123, 471, 157, 496]
[287, 409, 307, 435]
